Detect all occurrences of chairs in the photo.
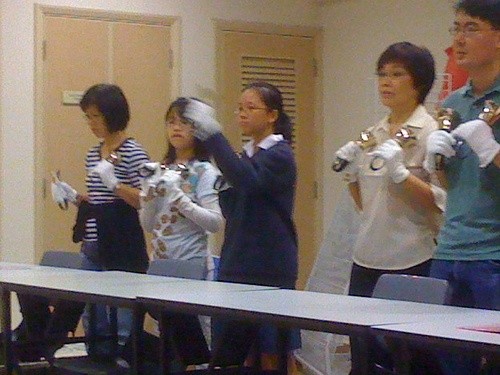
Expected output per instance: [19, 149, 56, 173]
[0, 252, 85, 366]
[369, 273, 453, 375]
[51, 259, 206, 375]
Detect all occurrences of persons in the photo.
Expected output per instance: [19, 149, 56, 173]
[333, 41, 448, 375]
[141, 98, 223, 375]
[50, 83, 148, 375]
[422, 0, 500, 375]
[182, 82, 301, 375]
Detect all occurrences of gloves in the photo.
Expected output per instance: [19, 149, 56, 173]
[366, 139, 410, 184]
[138, 162, 185, 203]
[181, 97, 222, 137]
[92, 158, 120, 191]
[422, 130, 456, 174]
[333, 140, 364, 183]
[51, 181, 78, 204]
[450, 118, 500, 168]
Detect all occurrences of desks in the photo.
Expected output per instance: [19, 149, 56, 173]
[0, 252, 500, 375]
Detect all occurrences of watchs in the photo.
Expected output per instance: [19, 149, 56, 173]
[114, 182, 121, 192]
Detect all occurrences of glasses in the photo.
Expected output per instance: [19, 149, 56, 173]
[234, 105, 271, 114]
[81, 112, 104, 121]
[448, 25, 500, 35]
[372, 71, 413, 80]
